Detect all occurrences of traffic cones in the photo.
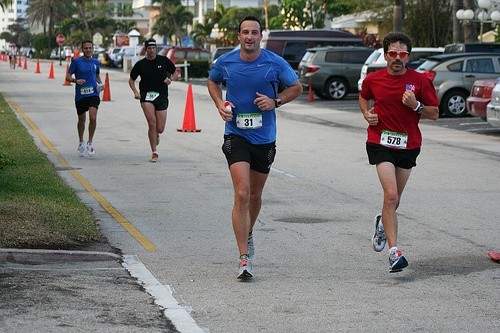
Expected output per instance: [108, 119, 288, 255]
[49, 62, 55, 78]
[305, 80, 316, 102]
[0, 52, 28, 69]
[176, 83, 202, 132]
[64, 65, 71, 85]
[35, 58, 41, 73]
[101, 73, 112, 101]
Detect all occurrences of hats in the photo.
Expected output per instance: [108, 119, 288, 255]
[145, 38, 156, 47]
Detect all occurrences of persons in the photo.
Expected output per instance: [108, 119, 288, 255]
[207, 16, 304, 278]
[1, 45, 17, 69]
[129, 38, 177, 162]
[65, 41, 105, 156]
[29, 50, 34, 59]
[66, 47, 72, 64]
[74, 47, 79, 57]
[358, 32, 439, 273]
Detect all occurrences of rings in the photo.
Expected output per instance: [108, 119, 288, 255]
[259, 106, 263, 108]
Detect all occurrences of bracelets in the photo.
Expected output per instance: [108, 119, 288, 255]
[412, 101, 424, 113]
[72, 79, 78, 83]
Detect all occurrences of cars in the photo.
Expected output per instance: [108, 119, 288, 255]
[49, 45, 213, 73]
[465, 76, 500, 129]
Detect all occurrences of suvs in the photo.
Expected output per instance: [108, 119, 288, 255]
[297, 46, 379, 100]
[358, 42, 500, 118]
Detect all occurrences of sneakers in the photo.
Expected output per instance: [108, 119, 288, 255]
[86, 141, 95, 155]
[156, 133, 159, 145]
[78, 141, 85, 157]
[237, 254, 253, 279]
[373, 214, 387, 252]
[488, 252, 500, 261]
[389, 250, 408, 273]
[150, 152, 159, 161]
[247, 231, 254, 257]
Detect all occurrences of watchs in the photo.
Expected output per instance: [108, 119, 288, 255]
[273, 98, 282, 108]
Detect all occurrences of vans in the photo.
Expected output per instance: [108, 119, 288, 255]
[260, 29, 364, 91]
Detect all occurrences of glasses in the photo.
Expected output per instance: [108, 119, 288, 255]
[386, 51, 409, 58]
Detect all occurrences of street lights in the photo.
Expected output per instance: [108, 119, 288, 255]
[455, 0, 500, 44]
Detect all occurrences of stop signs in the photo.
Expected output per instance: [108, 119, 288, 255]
[57, 34, 65, 45]
